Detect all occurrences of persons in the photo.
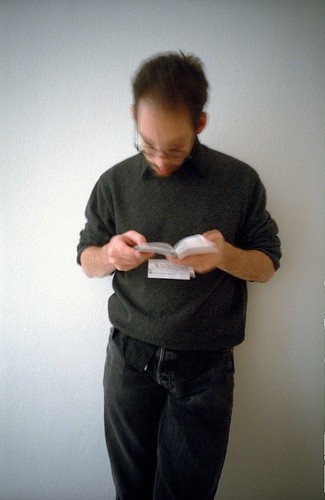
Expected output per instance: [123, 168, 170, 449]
[76, 53, 284, 500]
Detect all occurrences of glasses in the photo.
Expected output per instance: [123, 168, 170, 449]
[135, 115, 197, 161]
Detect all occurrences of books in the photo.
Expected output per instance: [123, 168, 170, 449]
[135, 234, 219, 258]
